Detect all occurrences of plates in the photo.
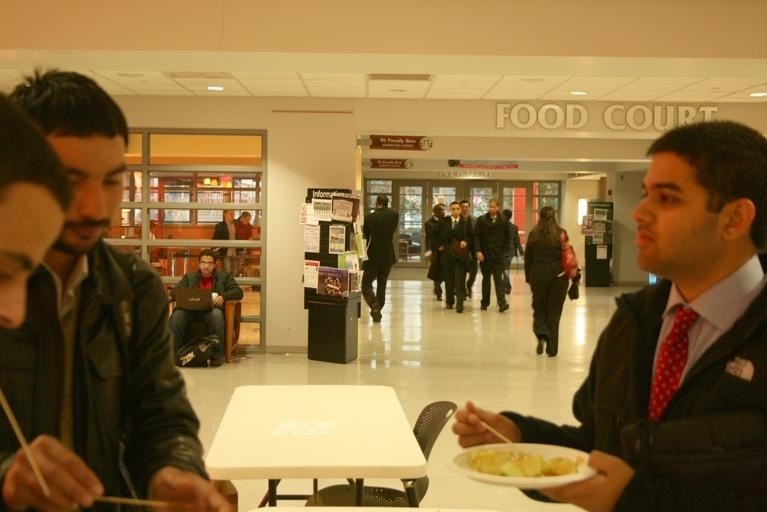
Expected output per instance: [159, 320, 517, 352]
[449, 444, 604, 492]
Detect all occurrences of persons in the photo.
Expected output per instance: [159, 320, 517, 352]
[361, 195, 400, 322]
[524, 206, 581, 357]
[248, 226, 260, 256]
[450, 121, 767, 512]
[212, 209, 239, 277]
[0, 92, 70, 330]
[168, 248, 244, 367]
[234, 211, 253, 255]
[0, 66, 236, 512]
[424, 199, 524, 313]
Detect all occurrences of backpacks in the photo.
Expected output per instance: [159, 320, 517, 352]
[176, 335, 219, 366]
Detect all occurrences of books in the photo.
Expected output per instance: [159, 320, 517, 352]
[317, 250, 364, 299]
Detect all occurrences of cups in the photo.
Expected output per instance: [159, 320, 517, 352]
[212, 292, 219, 302]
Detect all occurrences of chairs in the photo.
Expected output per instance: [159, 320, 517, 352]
[306, 400, 457, 506]
[224, 300, 241, 363]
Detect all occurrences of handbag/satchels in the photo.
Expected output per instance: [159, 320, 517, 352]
[560, 231, 577, 279]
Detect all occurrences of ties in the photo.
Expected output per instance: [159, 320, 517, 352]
[649, 309, 697, 421]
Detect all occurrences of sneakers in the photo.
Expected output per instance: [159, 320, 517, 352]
[481, 304, 508, 312]
[446, 304, 462, 312]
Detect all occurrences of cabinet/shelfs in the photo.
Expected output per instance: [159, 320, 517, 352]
[305, 188, 359, 361]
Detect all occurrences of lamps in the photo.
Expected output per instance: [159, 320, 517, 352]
[203, 176, 232, 188]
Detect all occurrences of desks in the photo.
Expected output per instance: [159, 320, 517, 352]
[204, 386, 428, 507]
